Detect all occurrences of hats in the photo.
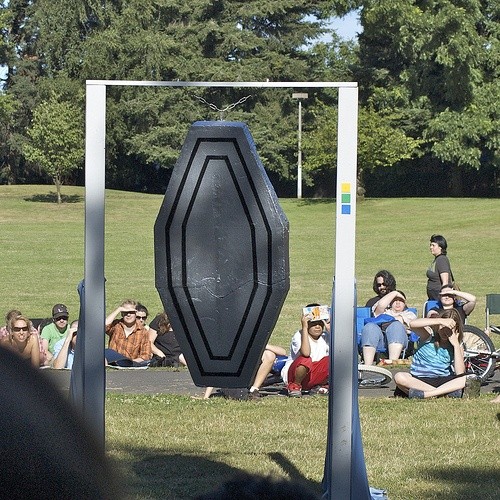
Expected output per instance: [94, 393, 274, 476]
[52, 304, 69, 318]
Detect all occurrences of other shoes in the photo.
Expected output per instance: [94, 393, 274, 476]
[250, 389, 260, 397]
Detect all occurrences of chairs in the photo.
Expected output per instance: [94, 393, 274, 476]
[486, 292, 500, 363]
[356, 299, 465, 365]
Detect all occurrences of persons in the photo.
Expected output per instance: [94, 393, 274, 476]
[361, 270, 476, 399]
[426, 235, 462, 301]
[280, 303, 331, 398]
[0, 300, 187, 370]
[189, 303, 288, 400]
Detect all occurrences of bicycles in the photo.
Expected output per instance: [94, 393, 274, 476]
[456, 326, 500, 384]
[356, 363, 393, 390]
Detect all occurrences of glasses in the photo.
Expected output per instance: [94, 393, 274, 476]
[12, 327, 29, 331]
[136, 316, 147, 320]
[56, 317, 68, 321]
[377, 283, 385, 287]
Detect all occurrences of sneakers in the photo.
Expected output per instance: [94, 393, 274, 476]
[288, 383, 301, 398]
[409, 388, 424, 399]
[448, 389, 462, 398]
[319, 387, 329, 394]
